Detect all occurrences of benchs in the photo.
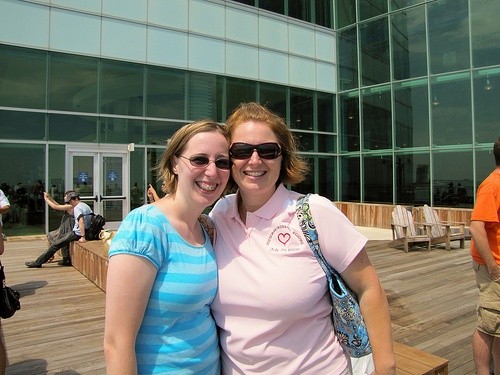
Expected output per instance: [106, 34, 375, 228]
[68, 239, 449, 375]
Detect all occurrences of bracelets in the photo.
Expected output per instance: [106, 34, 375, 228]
[81, 235, 86, 237]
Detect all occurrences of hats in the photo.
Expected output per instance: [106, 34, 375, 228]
[64, 191, 80, 203]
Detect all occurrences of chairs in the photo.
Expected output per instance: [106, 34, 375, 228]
[423, 204, 466, 251]
[389, 205, 434, 253]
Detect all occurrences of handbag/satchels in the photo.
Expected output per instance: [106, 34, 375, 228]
[0, 286, 21, 319]
[99, 229, 116, 257]
[296, 192, 371, 358]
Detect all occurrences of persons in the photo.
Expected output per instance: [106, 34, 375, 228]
[208, 101, 397, 375]
[102, 119, 233, 375]
[469, 136, 500, 375]
[24, 190, 94, 267]
[131, 182, 142, 208]
[0, 188, 11, 375]
[148, 181, 170, 201]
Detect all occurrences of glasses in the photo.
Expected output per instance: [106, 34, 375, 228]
[177, 154, 235, 170]
[230, 142, 281, 160]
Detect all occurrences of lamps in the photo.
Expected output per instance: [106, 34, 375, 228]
[433, 95, 439, 106]
[483, 74, 493, 93]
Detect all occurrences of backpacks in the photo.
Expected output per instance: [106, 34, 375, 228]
[77, 213, 106, 241]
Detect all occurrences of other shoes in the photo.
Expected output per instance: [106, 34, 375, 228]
[58, 257, 72, 266]
[25, 262, 42, 268]
